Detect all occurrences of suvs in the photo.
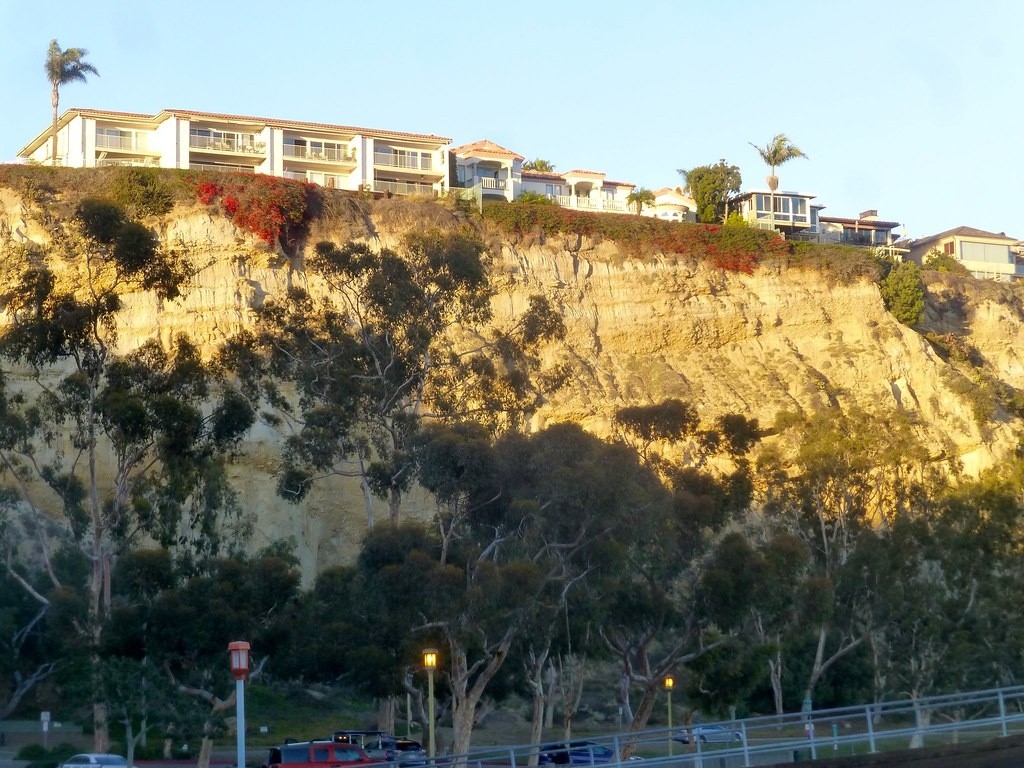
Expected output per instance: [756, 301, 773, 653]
[363, 737, 427, 765]
[266, 743, 388, 768]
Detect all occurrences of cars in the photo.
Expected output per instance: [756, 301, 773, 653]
[676, 723, 744, 744]
[537, 740, 645, 765]
[58, 754, 136, 767]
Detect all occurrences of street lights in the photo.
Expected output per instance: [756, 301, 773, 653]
[422, 648, 440, 768]
[228, 640, 251, 768]
[664, 672, 679, 760]
